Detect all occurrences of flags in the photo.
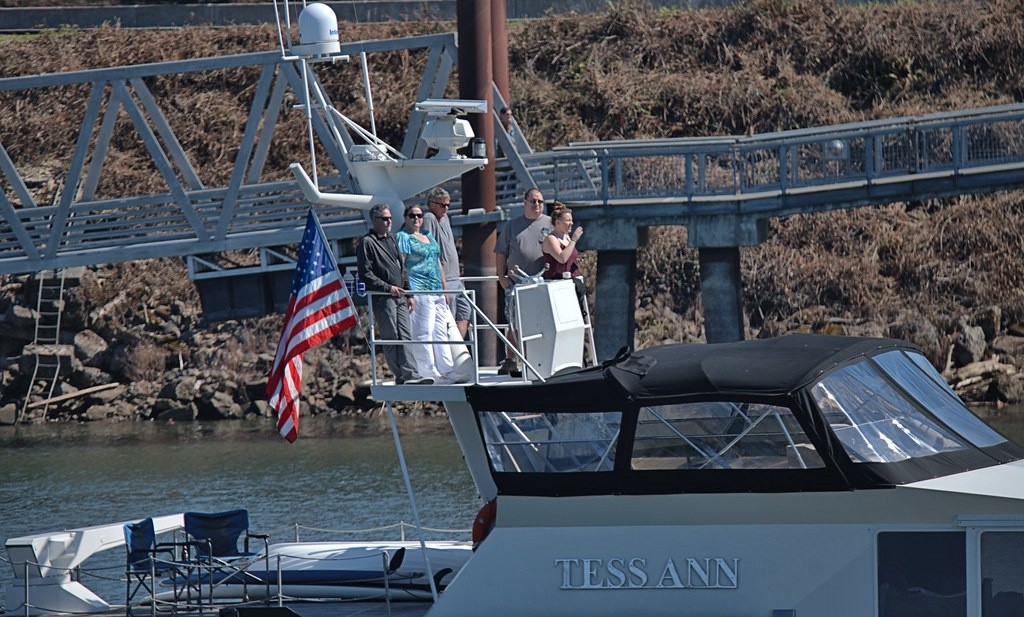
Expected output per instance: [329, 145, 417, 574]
[265, 207, 359, 445]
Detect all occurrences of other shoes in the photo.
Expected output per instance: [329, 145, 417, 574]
[425, 371, 470, 384]
[498, 357, 517, 375]
[396, 376, 435, 385]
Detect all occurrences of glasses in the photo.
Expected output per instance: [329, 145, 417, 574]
[406, 213, 424, 218]
[525, 198, 544, 204]
[432, 201, 450, 207]
[375, 216, 391, 221]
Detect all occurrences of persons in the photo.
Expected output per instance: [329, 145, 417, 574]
[355, 187, 472, 385]
[493, 188, 584, 375]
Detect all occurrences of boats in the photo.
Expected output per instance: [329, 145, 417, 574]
[0, 0, 1024, 617]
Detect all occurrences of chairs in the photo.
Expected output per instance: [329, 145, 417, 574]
[123, 517, 202, 617]
[183, 509, 270, 617]
